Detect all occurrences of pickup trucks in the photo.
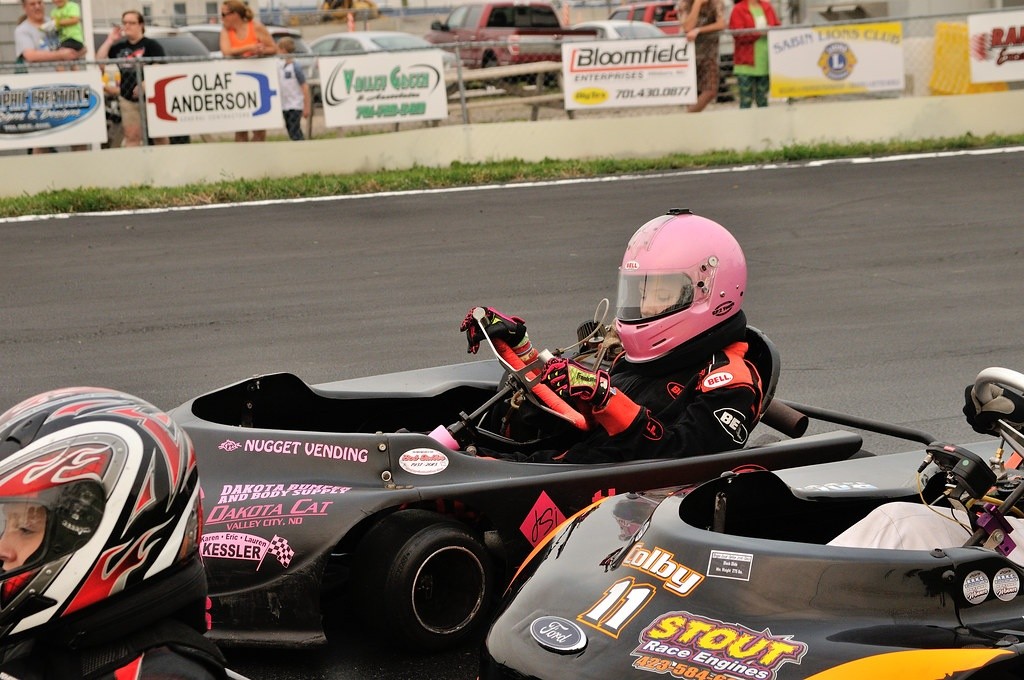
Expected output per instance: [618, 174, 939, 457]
[608, 1, 738, 56]
[423, 0, 598, 91]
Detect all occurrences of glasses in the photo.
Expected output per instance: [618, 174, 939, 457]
[121, 20, 139, 25]
[221, 10, 235, 18]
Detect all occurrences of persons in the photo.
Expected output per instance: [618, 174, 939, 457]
[461, 212, 763, 498]
[0, 387, 224, 680]
[277, 37, 311, 141]
[815, 383, 1024, 569]
[679, 0, 726, 113]
[14, 0, 90, 155]
[96, 11, 166, 147]
[718, 0, 780, 110]
[219, 0, 279, 142]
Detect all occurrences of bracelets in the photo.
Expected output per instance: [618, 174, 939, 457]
[694, 28, 700, 33]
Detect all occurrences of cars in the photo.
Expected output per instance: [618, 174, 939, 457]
[560, 20, 667, 41]
[310, 30, 462, 103]
[93, 25, 215, 117]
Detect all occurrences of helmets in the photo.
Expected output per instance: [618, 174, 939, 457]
[614, 207, 749, 364]
[1, 385, 207, 680]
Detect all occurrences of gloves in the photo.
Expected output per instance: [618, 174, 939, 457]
[457, 305, 528, 354]
[959, 380, 1024, 440]
[542, 358, 614, 411]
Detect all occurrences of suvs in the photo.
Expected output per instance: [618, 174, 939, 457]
[178, 25, 317, 100]
